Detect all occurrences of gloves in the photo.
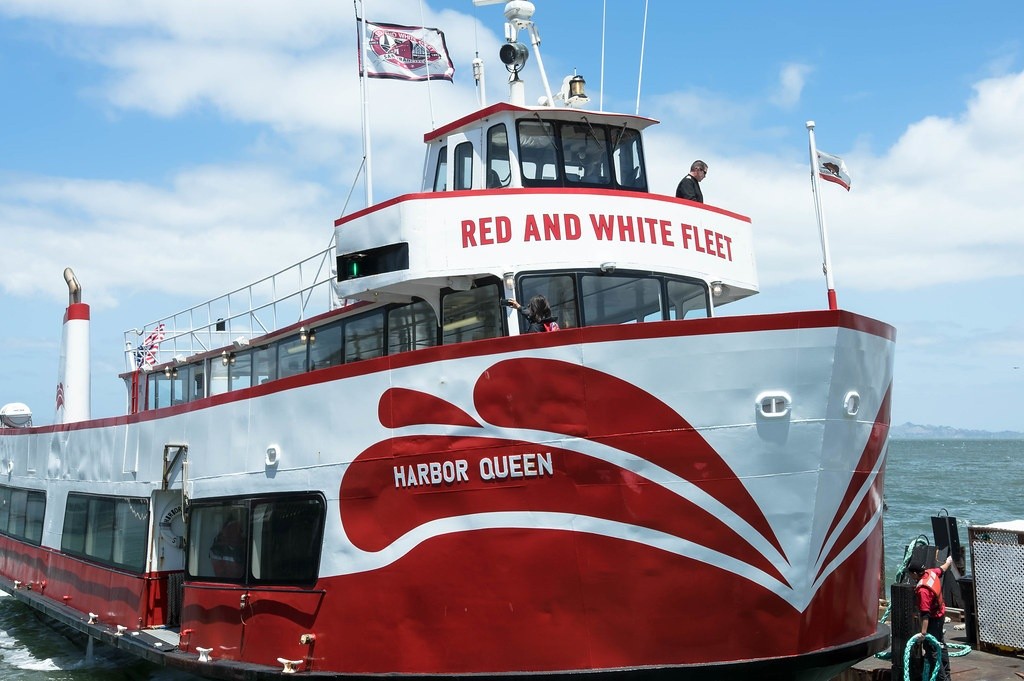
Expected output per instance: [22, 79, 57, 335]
[918, 634, 925, 643]
[946, 556, 953, 565]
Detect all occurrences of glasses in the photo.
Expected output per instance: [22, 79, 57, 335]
[701, 169, 707, 174]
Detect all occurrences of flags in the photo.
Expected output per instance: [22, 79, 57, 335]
[811, 149, 851, 191]
[358, 18, 456, 85]
[134, 323, 164, 373]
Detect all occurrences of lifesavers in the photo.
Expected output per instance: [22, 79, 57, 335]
[160, 505, 184, 547]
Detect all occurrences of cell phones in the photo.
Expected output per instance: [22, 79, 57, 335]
[500, 299, 513, 306]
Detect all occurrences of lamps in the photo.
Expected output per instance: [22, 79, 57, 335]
[504, 272, 516, 290]
[222, 351, 229, 366]
[165, 366, 170, 380]
[309, 329, 316, 345]
[173, 368, 178, 380]
[287, 342, 318, 354]
[500, 42, 529, 81]
[230, 352, 236, 366]
[473, 63, 481, 86]
[443, 311, 486, 331]
[712, 281, 723, 297]
[299, 327, 308, 345]
[569, 75, 588, 98]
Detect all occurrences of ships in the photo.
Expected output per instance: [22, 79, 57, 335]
[0, 0, 904, 681]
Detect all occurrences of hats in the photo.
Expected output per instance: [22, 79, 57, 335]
[903, 560, 925, 574]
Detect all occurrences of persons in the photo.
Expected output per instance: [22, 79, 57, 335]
[508, 295, 558, 333]
[676, 160, 708, 203]
[908, 555, 953, 681]
[488, 168, 502, 187]
